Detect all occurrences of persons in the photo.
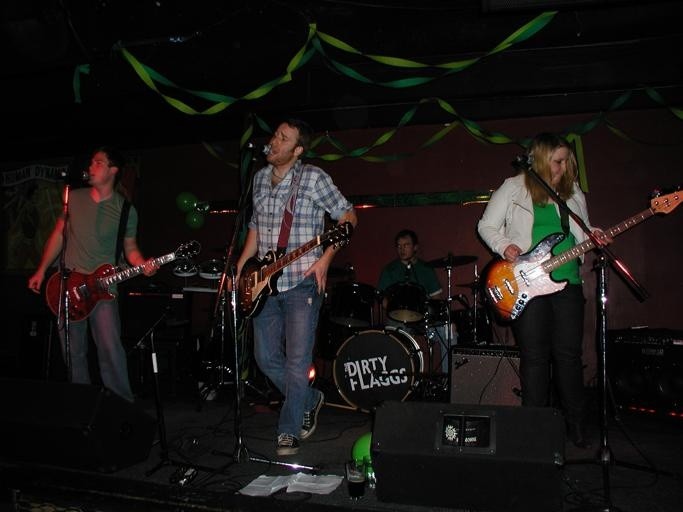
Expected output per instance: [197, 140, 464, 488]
[479, 132, 613, 449]
[227, 122, 358, 456]
[460, 305, 530, 351]
[29, 151, 160, 402]
[375, 229, 456, 375]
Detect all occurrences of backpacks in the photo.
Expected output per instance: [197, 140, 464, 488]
[199, 245, 225, 279]
[328, 281, 377, 327]
[426, 299, 449, 327]
[170, 242, 198, 277]
[386, 282, 425, 322]
[332, 326, 424, 413]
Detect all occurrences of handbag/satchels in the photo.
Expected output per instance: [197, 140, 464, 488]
[130, 255, 141, 265]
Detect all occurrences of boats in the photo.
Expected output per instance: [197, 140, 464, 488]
[606, 329, 683, 415]
[0, 381, 155, 475]
[372, 400, 569, 512]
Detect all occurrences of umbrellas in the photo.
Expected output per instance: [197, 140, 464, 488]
[574, 439, 592, 448]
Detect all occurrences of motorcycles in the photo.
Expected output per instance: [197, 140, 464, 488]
[196, 287, 269, 410]
[510, 168, 683, 511]
[192, 155, 323, 484]
[124, 313, 230, 476]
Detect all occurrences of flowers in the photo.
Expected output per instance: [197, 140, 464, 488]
[164, 299, 177, 312]
[246, 140, 271, 157]
[515, 151, 535, 164]
[53, 169, 90, 183]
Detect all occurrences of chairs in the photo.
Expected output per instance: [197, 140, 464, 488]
[300, 390, 324, 439]
[276, 432, 300, 456]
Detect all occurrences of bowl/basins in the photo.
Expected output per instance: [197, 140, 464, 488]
[272, 170, 284, 180]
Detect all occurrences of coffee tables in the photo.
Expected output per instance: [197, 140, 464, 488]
[456, 281, 482, 288]
[426, 255, 478, 268]
